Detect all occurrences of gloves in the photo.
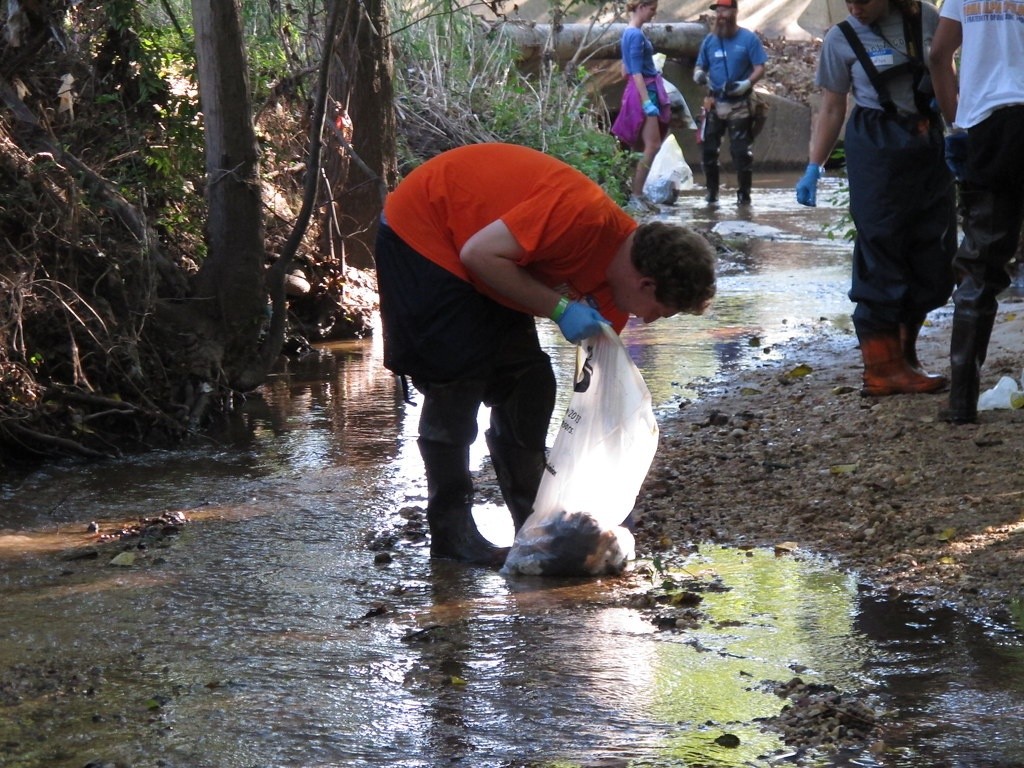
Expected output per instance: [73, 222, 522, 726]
[944, 130, 968, 183]
[556, 300, 613, 345]
[728, 79, 752, 97]
[796, 163, 825, 206]
[641, 99, 659, 117]
[692, 66, 705, 84]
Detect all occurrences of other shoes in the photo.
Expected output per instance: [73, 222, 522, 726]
[628, 192, 660, 214]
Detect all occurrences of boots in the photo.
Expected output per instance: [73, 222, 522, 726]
[416, 435, 513, 567]
[851, 315, 947, 396]
[937, 313, 995, 425]
[737, 169, 752, 206]
[486, 428, 544, 539]
[701, 162, 719, 202]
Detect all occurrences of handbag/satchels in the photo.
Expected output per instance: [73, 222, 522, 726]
[695, 106, 707, 145]
[744, 87, 770, 136]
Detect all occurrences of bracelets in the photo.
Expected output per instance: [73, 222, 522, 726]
[549, 294, 569, 321]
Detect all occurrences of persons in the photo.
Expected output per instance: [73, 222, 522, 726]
[373, 141, 719, 569]
[621, 0, 671, 215]
[692, 1, 766, 207]
[794, 1, 1024, 425]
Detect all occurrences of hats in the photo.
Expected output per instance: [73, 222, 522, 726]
[709, 0, 737, 10]
